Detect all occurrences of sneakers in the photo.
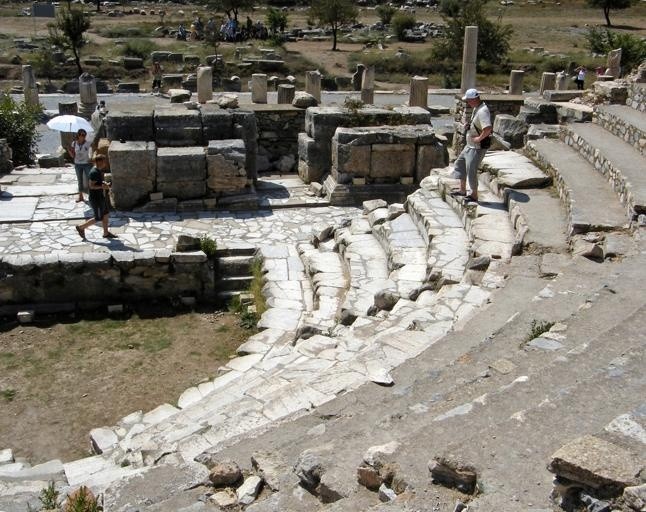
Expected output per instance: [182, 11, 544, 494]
[450, 192, 466, 197]
[463, 196, 478, 201]
[103, 233, 118, 238]
[76, 225, 85, 239]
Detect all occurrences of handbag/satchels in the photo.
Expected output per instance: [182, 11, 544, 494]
[481, 137, 492, 149]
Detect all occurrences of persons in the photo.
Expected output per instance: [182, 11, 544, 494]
[449, 88, 492, 202]
[76, 154, 119, 239]
[178, 17, 284, 41]
[150, 62, 162, 96]
[67, 129, 91, 202]
[352, 64, 365, 91]
[574, 64, 585, 90]
[596, 66, 603, 75]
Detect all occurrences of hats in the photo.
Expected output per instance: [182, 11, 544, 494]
[461, 89, 480, 100]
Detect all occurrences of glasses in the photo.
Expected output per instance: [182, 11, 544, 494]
[78, 133, 86, 137]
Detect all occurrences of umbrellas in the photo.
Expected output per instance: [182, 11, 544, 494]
[47, 113, 94, 146]
